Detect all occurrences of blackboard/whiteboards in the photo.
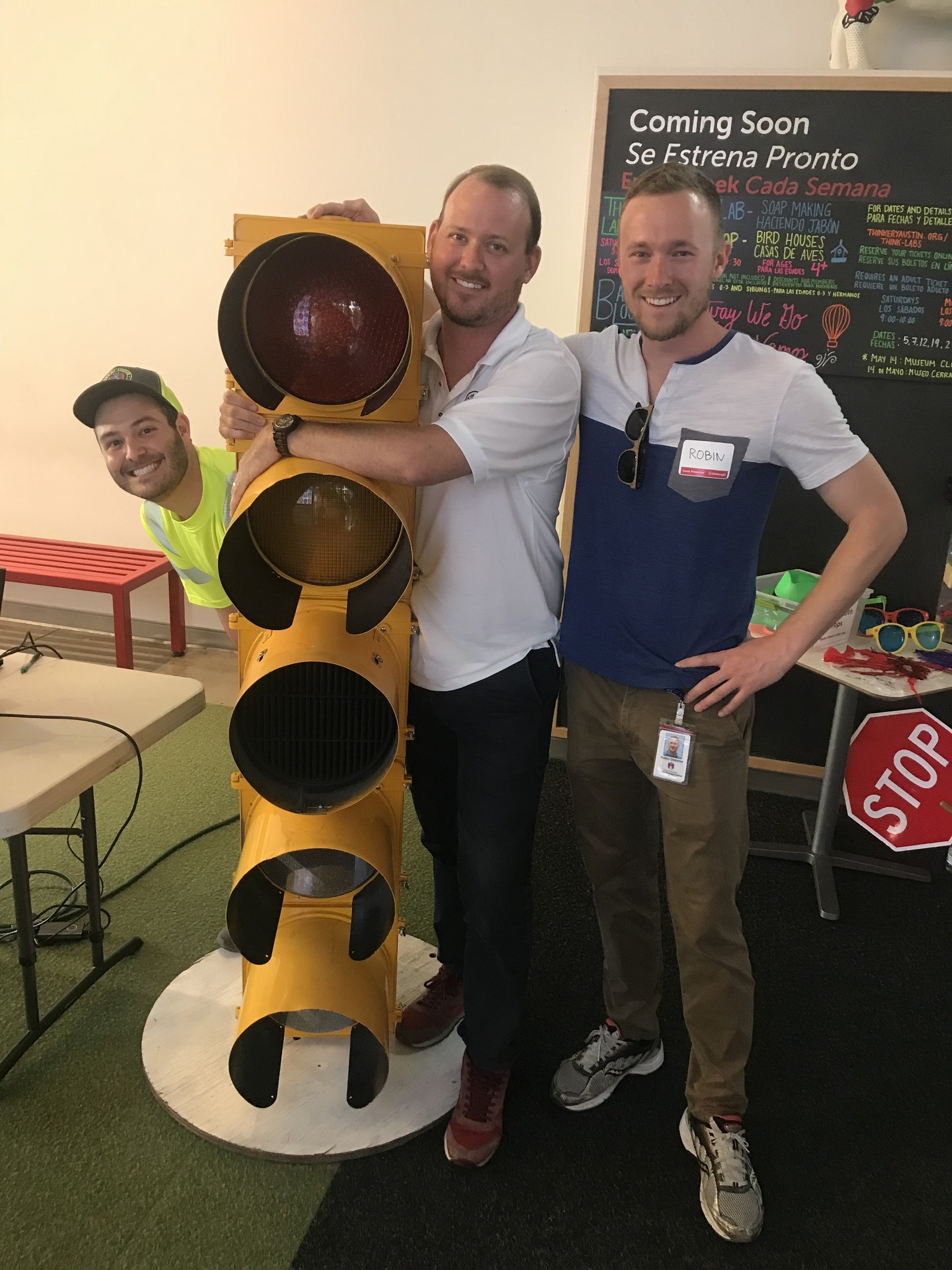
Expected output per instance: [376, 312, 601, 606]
[549, 77, 952, 778]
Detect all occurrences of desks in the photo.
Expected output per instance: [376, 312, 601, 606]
[744, 632, 952, 920]
[0, 644, 206, 1080]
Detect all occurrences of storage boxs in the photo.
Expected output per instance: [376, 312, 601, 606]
[745, 568, 874, 652]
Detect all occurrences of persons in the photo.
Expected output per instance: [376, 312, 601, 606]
[216, 166, 583, 1170]
[295, 162, 907, 1243]
[663, 735, 683, 759]
[73, 365, 237, 652]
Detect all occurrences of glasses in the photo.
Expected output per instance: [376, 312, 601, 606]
[617, 402, 653, 490]
[857, 607, 928, 638]
[865, 621, 944, 654]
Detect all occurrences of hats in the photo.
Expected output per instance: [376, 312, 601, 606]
[74, 365, 185, 428]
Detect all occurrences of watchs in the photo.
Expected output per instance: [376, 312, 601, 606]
[272, 414, 305, 459]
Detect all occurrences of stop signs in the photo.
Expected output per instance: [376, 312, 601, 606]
[844, 710, 952, 854]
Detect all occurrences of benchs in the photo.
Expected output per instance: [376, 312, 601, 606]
[0, 534, 187, 668]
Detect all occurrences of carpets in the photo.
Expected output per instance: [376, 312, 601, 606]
[289, 753, 951, 1270]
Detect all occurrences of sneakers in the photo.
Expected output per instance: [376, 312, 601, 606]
[679, 1106, 764, 1243]
[444, 1049, 508, 1167]
[394, 964, 463, 1048]
[551, 1018, 664, 1112]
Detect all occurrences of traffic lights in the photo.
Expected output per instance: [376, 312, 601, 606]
[223, 214, 427, 1112]
